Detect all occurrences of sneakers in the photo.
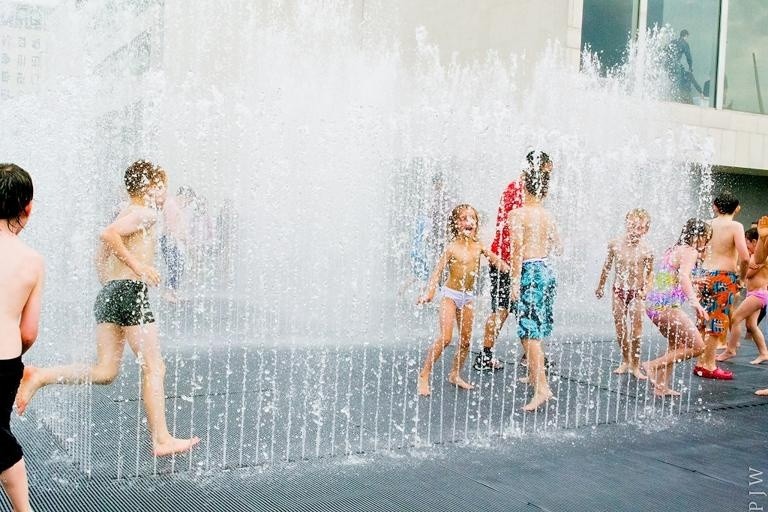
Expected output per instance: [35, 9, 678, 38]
[518, 353, 557, 370]
[473, 351, 504, 371]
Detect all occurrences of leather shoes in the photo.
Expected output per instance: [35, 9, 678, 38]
[694, 366, 734, 380]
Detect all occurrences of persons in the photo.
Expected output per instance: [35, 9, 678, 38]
[16, 157, 201, 458]
[506, 167, 562, 412]
[643, 217, 714, 398]
[396, 170, 456, 304]
[412, 202, 511, 397]
[107, 184, 130, 225]
[594, 207, 656, 381]
[691, 190, 751, 380]
[714, 214, 768, 396]
[160, 184, 238, 303]
[472, 152, 556, 374]
[0, 164, 46, 511]
